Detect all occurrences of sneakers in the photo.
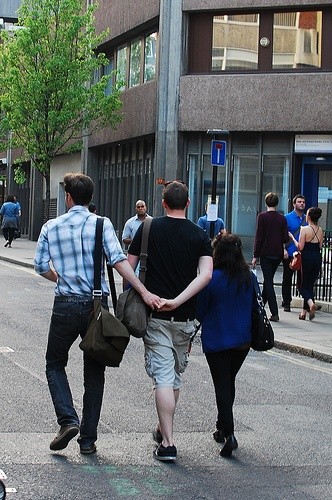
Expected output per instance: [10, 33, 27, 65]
[49, 424, 80, 453]
[78, 440, 97, 454]
[151, 426, 163, 442]
[153, 442, 178, 461]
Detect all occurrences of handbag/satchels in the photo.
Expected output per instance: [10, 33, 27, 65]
[251, 292, 275, 352]
[115, 287, 148, 339]
[76, 306, 131, 368]
[288, 243, 302, 271]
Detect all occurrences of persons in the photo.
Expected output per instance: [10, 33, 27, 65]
[194, 233, 261, 456]
[251, 192, 289, 322]
[34, 171, 163, 455]
[0, 195, 21, 248]
[123, 181, 214, 461]
[288, 206, 325, 321]
[282, 195, 322, 312]
[87, 203, 97, 214]
[121, 199, 152, 279]
[197, 202, 227, 239]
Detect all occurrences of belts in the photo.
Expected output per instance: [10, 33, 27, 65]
[152, 312, 196, 322]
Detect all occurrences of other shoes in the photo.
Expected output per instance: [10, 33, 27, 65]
[3, 240, 11, 248]
[213, 424, 239, 458]
[269, 314, 280, 322]
[308, 304, 316, 321]
[298, 312, 307, 320]
[284, 305, 291, 311]
